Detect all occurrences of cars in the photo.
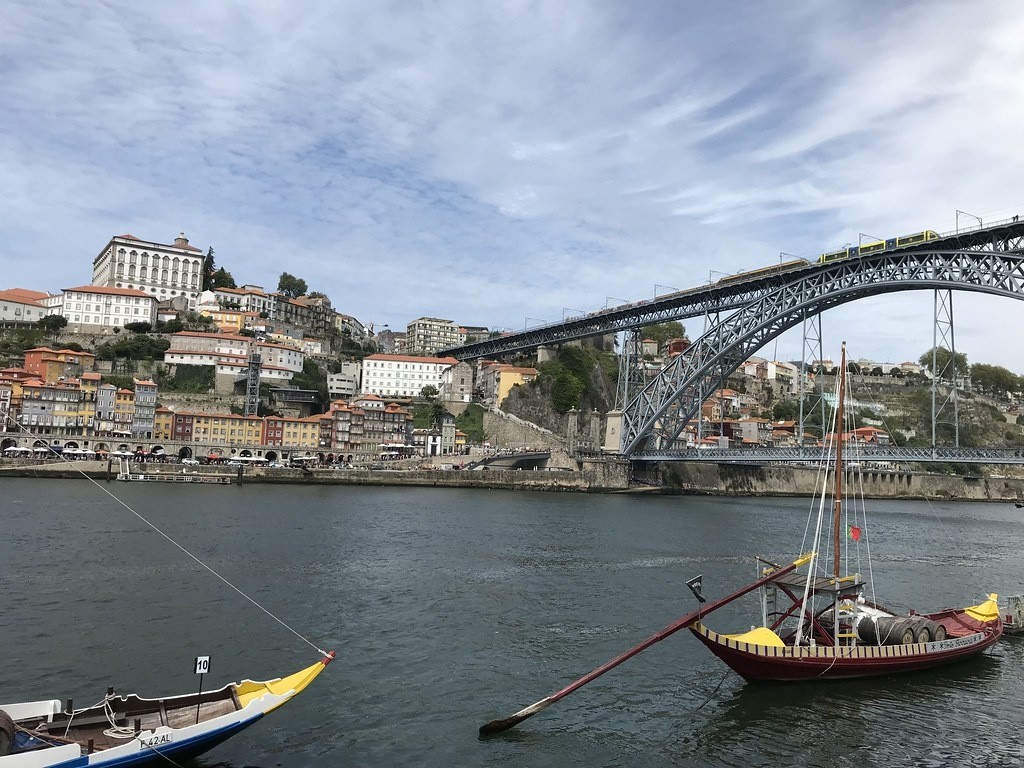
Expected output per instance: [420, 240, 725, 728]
[227, 461, 241, 466]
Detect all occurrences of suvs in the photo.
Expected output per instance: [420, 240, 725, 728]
[182, 457, 199, 465]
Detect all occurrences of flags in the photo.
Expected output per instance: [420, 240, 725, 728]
[685, 574, 707, 603]
[847, 524, 862, 541]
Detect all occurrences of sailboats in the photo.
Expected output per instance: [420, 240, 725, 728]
[0, 405, 335, 768]
[688, 340, 1003, 683]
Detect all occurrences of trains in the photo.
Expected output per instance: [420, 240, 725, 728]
[564, 230, 941, 323]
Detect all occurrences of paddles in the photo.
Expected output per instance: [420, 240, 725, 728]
[477, 553, 818, 740]
